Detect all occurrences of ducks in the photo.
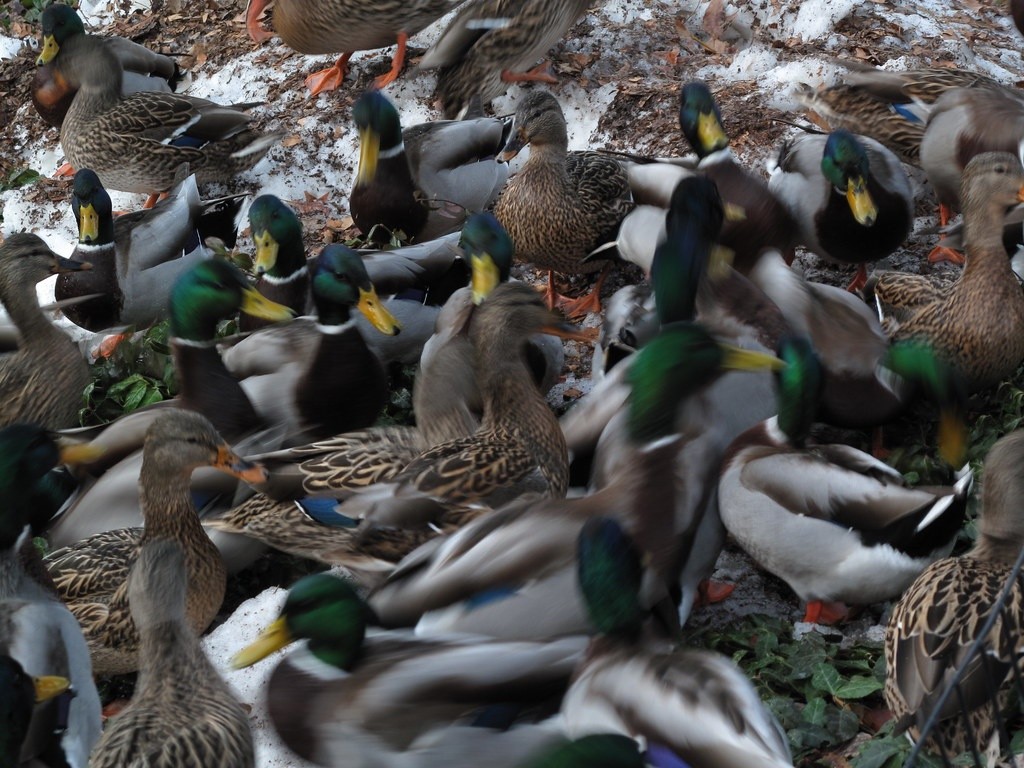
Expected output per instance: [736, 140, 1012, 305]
[0, 0, 1024, 768]
